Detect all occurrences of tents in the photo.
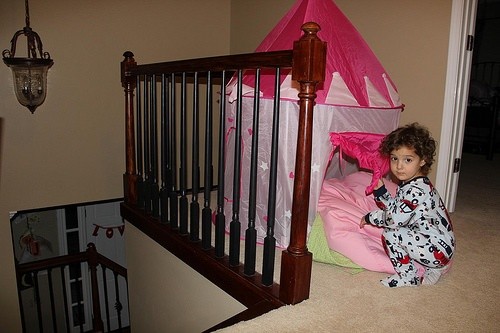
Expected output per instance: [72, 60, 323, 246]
[224, 1, 404, 271]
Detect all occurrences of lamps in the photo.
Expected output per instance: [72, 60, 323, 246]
[1, 0, 54, 113]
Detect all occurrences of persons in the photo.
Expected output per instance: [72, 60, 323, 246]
[359, 122, 455, 290]
